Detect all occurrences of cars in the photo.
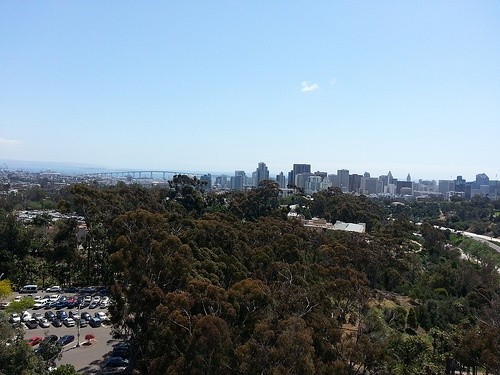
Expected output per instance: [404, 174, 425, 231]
[26, 336, 42, 347]
[39, 335, 58, 347]
[55, 335, 75, 347]
[108, 341, 129, 357]
[14, 295, 23, 303]
[39, 318, 50, 328]
[70, 311, 81, 320]
[95, 286, 103, 291]
[52, 318, 62, 327]
[63, 317, 75, 327]
[78, 319, 87, 328]
[32, 312, 42, 320]
[95, 312, 108, 320]
[81, 312, 92, 320]
[1, 301, 12, 308]
[45, 311, 55, 320]
[64, 287, 78, 293]
[28, 295, 112, 310]
[105, 356, 129, 367]
[10, 310, 37, 332]
[55, 310, 68, 320]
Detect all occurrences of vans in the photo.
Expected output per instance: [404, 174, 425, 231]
[19, 284, 38, 293]
[88, 317, 101, 327]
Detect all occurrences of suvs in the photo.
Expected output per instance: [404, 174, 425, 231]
[46, 285, 61, 293]
[80, 286, 96, 293]
